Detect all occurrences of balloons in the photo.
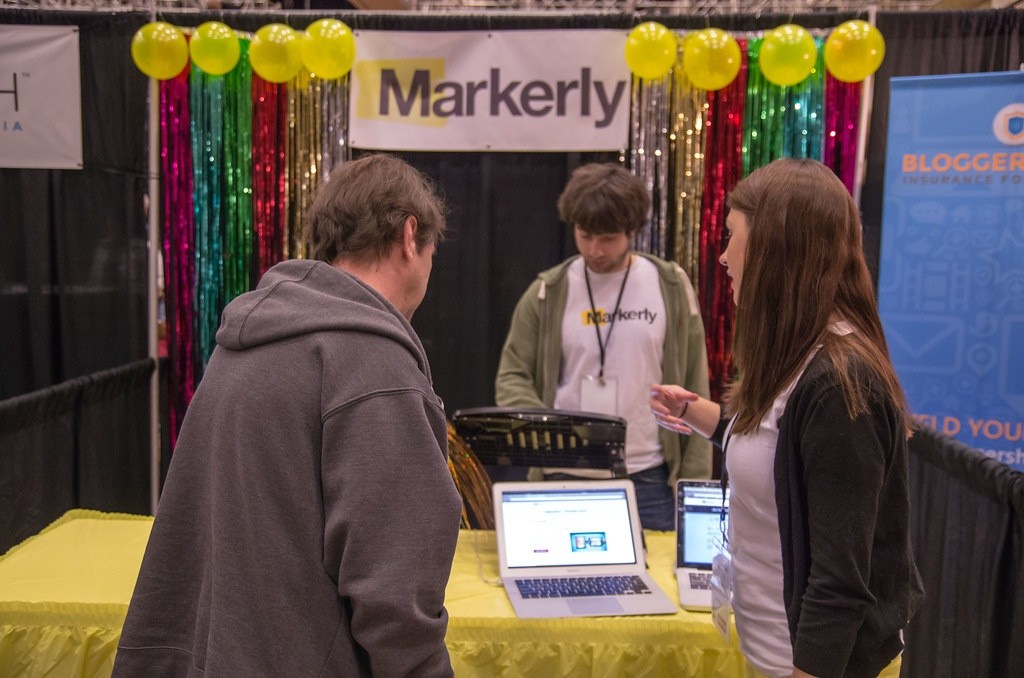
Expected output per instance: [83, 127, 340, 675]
[131, 18, 356, 83]
[625, 18, 882, 90]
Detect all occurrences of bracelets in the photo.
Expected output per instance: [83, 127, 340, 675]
[680, 401, 690, 419]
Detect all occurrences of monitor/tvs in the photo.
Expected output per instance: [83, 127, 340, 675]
[451, 406, 629, 483]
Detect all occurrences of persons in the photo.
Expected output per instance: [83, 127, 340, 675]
[649, 158, 924, 678]
[494, 162, 710, 532]
[109, 154, 463, 678]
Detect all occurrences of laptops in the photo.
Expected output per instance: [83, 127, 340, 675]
[672, 478, 737, 615]
[493, 480, 677, 618]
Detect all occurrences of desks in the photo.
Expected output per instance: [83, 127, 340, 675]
[0, 508, 903, 678]
